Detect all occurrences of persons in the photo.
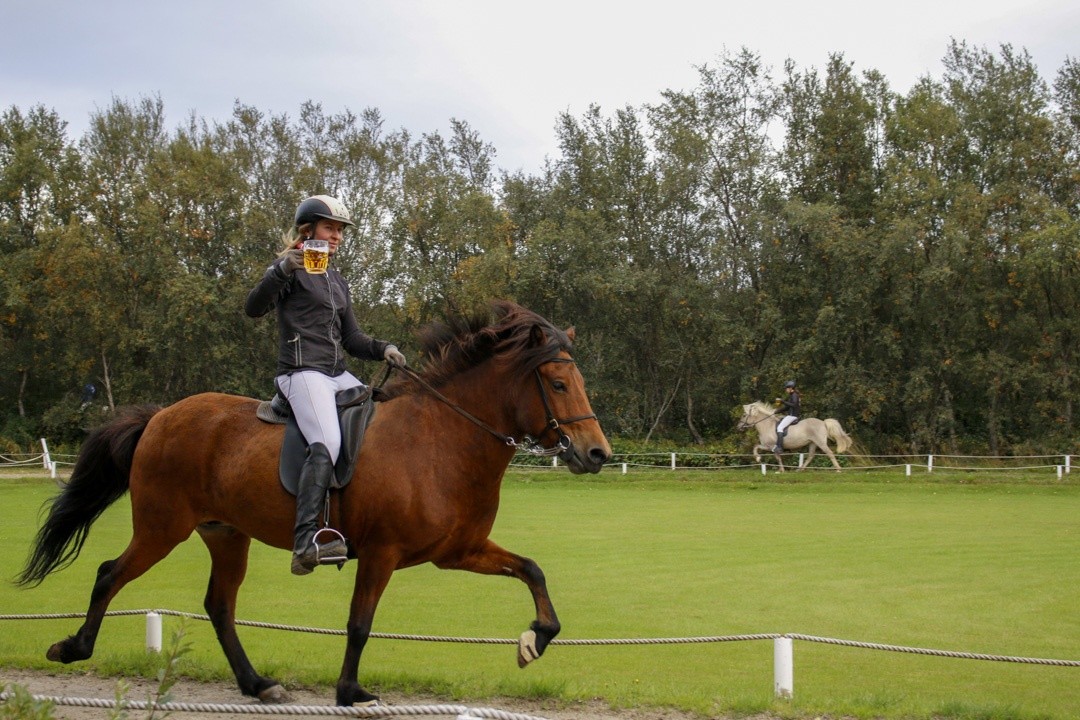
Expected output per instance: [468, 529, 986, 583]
[245, 195, 406, 576]
[77, 381, 96, 415]
[774, 381, 802, 452]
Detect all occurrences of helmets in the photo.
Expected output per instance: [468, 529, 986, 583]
[294, 194, 357, 229]
[784, 381, 796, 387]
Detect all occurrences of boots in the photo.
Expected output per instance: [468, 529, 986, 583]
[773, 432, 784, 452]
[290, 442, 348, 576]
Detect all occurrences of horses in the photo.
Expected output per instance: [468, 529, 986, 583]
[1, 286, 616, 708]
[735, 398, 854, 474]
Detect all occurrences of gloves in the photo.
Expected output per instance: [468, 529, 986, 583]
[384, 345, 407, 369]
[280, 248, 304, 276]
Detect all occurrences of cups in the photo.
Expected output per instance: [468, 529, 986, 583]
[303, 240, 329, 275]
[776, 398, 782, 405]
